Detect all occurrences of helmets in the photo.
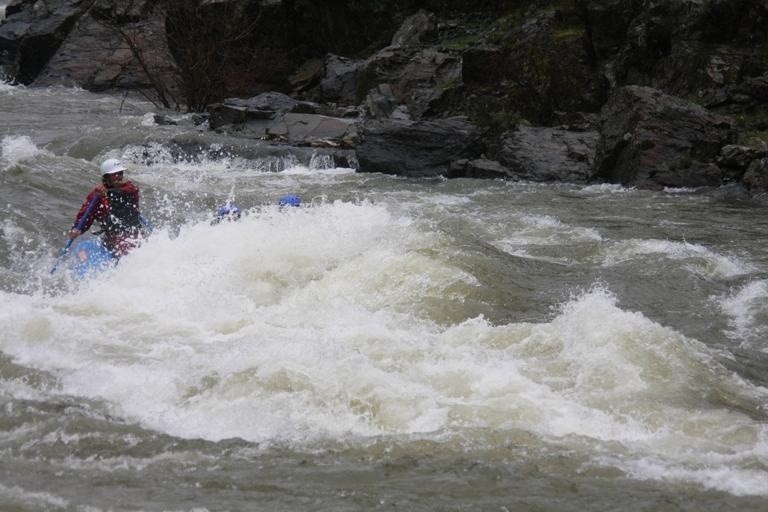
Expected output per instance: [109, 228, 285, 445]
[99, 158, 127, 176]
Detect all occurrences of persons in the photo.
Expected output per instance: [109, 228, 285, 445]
[70, 158, 141, 259]
